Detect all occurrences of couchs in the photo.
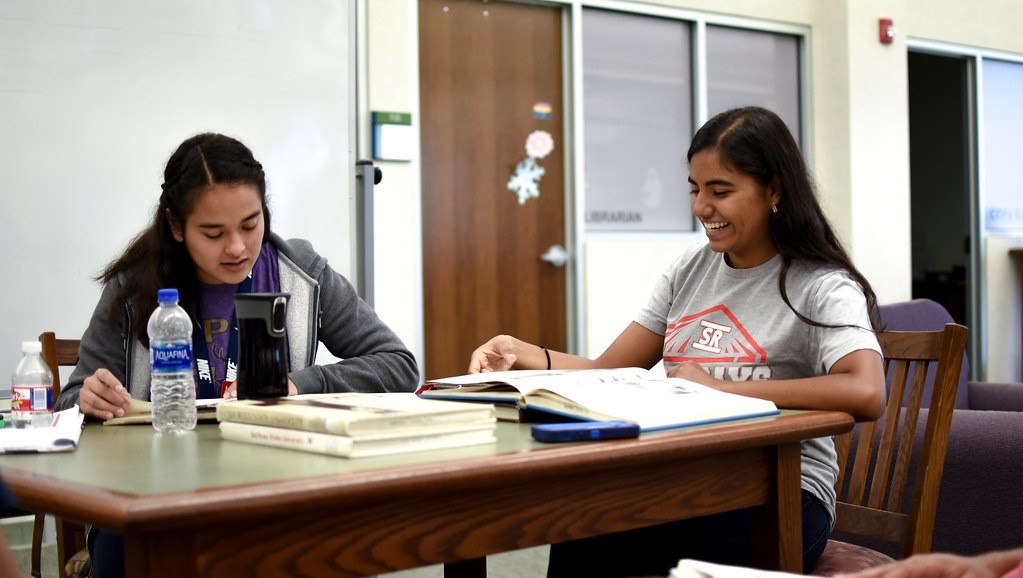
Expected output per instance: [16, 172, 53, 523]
[839, 297, 1023, 556]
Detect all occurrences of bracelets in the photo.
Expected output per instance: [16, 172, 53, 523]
[539, 346, 551, 370]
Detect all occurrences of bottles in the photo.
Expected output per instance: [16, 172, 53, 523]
[147, 289, 198, 433]
[11, 341, 54, 429]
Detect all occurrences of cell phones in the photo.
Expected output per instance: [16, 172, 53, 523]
[531, 421, 640, 442]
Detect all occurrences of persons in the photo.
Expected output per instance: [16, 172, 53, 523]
[468, 107, 887, 578]
[54, 132, 420, 578]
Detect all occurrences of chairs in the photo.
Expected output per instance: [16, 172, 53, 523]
[806, 319, 970, 578]
[39, 331, 94, 578]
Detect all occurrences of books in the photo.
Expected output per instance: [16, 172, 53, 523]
[415, 367, 781, 433]
[103, 398, 238, 427]
[217, 393, 498, 459]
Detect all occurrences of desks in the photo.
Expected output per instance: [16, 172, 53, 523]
[0, 390, 854, 578]
